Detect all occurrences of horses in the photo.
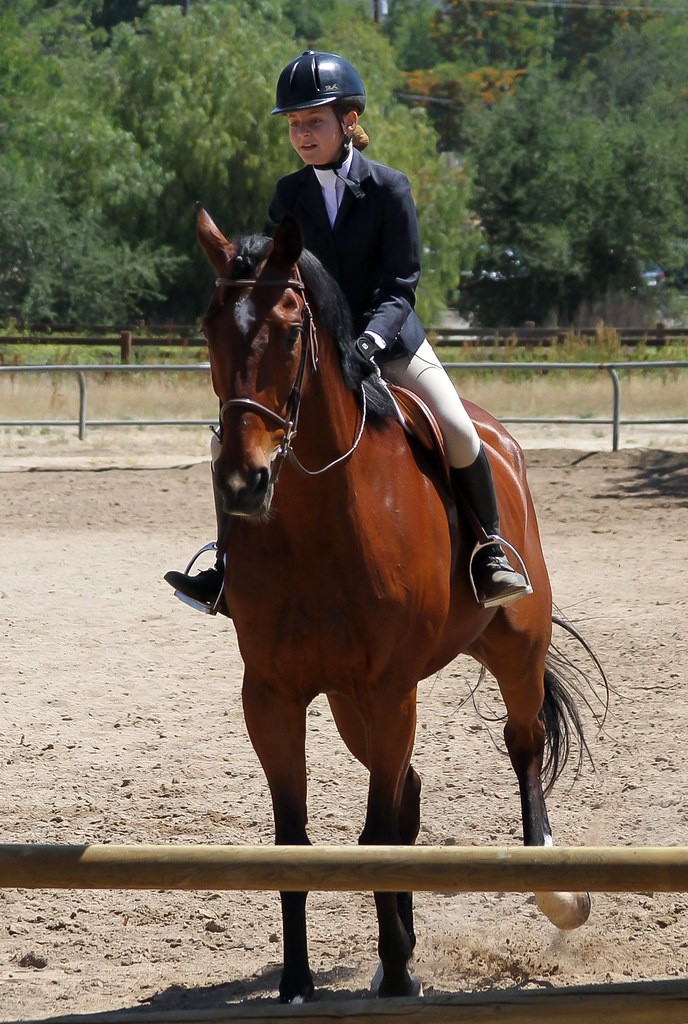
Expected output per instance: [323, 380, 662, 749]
[185, 200, 615, 1009]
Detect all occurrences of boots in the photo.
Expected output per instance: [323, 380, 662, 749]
[448, 446, 527, 603]
[163, 461, 228, 618]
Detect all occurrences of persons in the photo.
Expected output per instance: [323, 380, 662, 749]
[164, 51, 528, 619]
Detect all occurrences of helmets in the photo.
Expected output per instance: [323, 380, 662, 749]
[271, 51, 365, 115]
